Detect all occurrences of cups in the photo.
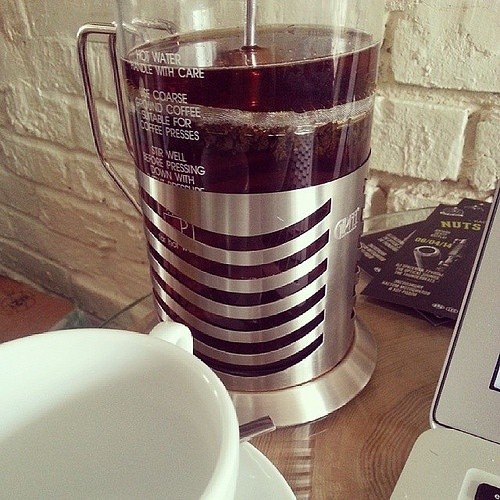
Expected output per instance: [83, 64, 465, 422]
[76, 0, 388, 429]
[1, 322, 240, 500]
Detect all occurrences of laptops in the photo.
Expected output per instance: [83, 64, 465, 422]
[389, 178, 500, 500]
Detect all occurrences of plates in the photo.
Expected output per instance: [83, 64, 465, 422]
[237, 440, 298, 500]
